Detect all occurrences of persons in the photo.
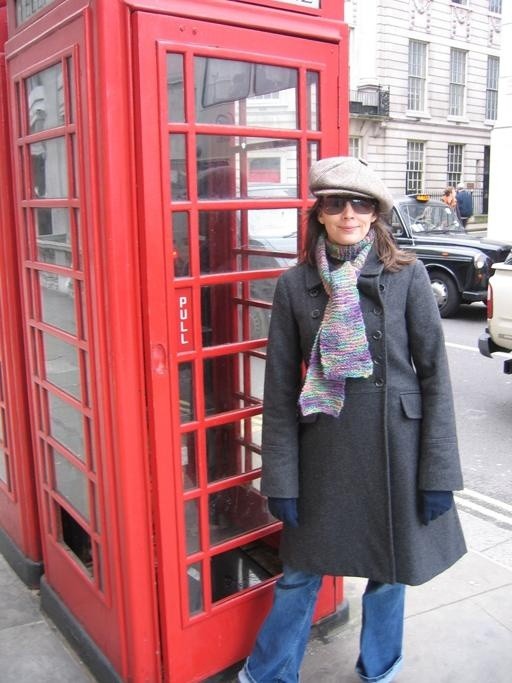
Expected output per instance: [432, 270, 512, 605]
[225, 152, 468, 683]
[453, 182, 474, 229]
[437, 185, 457, 220]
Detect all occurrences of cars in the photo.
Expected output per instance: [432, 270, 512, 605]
[391, 196, 512, 375]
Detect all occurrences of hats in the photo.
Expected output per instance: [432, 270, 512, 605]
[308, 156, 394, 214]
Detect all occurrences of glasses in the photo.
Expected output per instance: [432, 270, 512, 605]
[317, 198, 376, 214]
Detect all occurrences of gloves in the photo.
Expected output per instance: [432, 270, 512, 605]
[418, 491, 453, 526]
[268, 498, 299, 529]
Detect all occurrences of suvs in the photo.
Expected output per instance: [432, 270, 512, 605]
[236, 182, 315, 351]
